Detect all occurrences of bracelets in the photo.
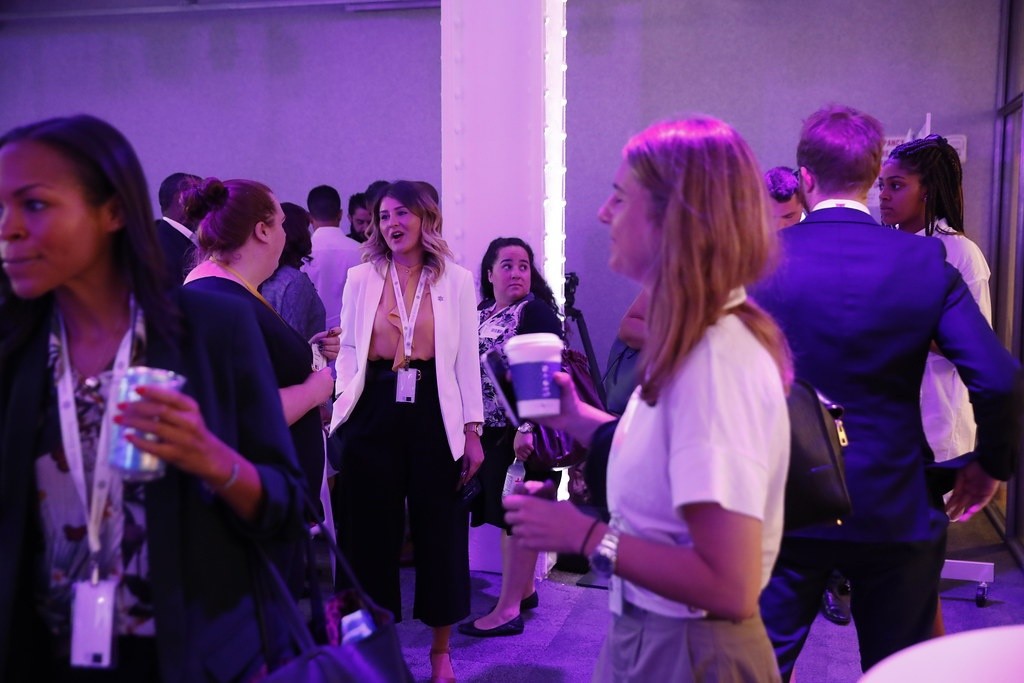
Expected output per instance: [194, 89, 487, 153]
[580, 518, 601, 555]
[216, 462, 239, 490]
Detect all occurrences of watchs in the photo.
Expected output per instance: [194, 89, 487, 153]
[464, 424, 483, 437]
[589, 526, 622, 577]
[518, 422, 532, 433]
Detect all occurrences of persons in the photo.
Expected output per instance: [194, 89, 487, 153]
[509, 116, 793, 683]
[759, 105, 1024, 683]
[458, 237, 566, 636]
[0, 114, 486, 683]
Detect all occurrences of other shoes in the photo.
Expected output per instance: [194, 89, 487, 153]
[457, 614, 524, 636]
[491, 590, 540, 609]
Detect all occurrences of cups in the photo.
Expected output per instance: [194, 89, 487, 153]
[99, 367, 187, 482]
[504, 333, 564, 418]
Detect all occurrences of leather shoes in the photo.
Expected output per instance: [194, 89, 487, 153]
[820, 589, 851, 624]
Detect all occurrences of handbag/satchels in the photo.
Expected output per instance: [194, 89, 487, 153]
[531, 349, 606, 467]
[255, 461, 415, 683]
[583, 379, 853, 532]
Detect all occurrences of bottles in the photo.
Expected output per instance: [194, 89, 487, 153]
[501, 458, 526, 497]
